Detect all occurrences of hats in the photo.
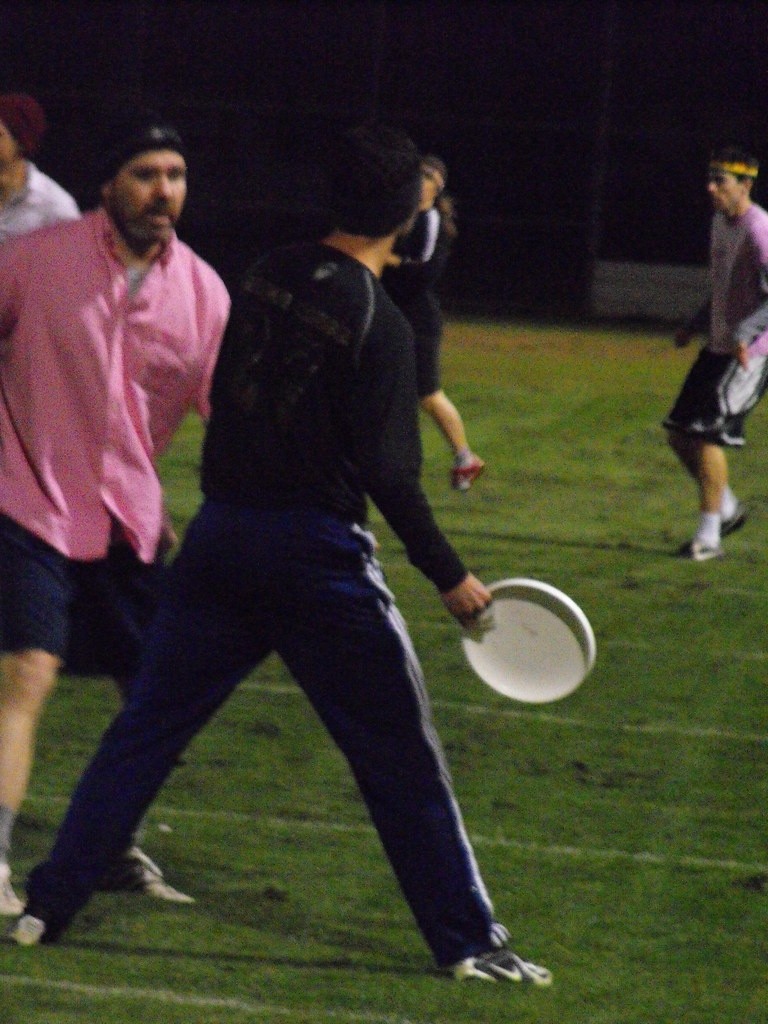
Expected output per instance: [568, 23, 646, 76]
[87, 108, 191, 195]
[318, 115, 428, 232]
[2, 90, 48, 162]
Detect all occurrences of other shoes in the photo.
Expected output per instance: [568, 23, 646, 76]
[452, 453, 485, 490]
[668, 539, 726, 562]
[715, 501, 752, 537]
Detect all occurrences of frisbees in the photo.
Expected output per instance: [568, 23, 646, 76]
[456, 577, 598, 706]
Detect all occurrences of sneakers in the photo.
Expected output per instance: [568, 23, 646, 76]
[2, 913, 46, 947]
[0, 862, 26, 918]
[452, 946, 554, 988]
[96, 844, 195, 906]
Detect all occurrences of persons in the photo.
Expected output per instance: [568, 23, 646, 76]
[661, 152, 767, 561]
[2, 91, 557, 987]
